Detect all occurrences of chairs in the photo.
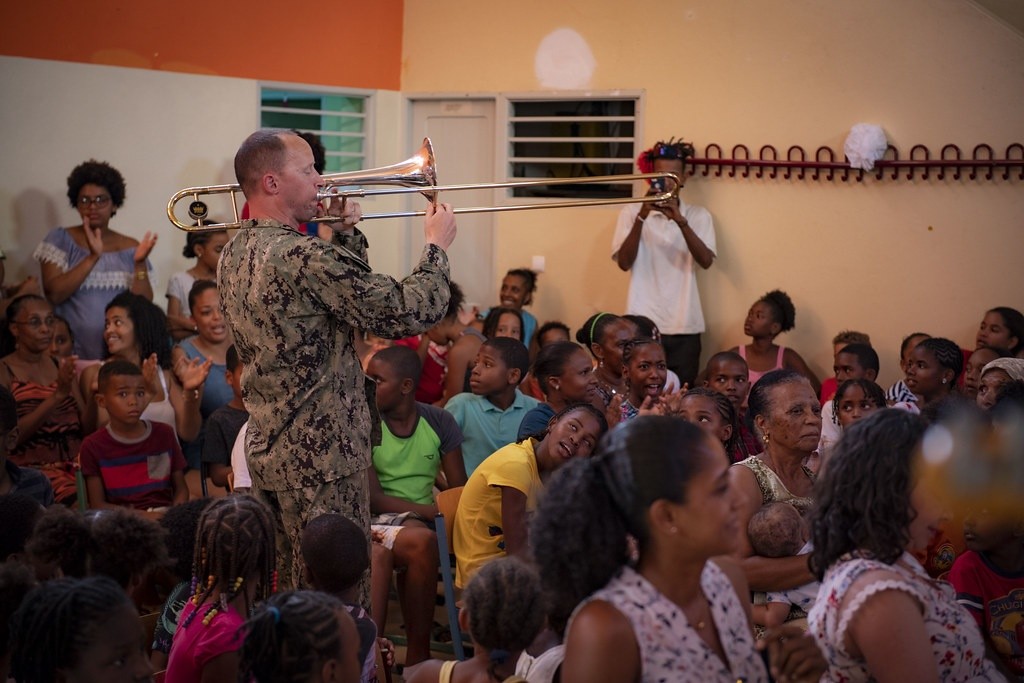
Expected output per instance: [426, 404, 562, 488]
[436, 486, 475, 661]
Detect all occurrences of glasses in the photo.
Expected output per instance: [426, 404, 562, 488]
[16, 317, 56, 329]
[75, 195, 113, 210]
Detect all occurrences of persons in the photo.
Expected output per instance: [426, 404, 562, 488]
[216, 130, 457, 618]
[0, 126, 1024, 683]
[610, 136, 716, 391]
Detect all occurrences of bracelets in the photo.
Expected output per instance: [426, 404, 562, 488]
[678, 218, 687, 227]
[182, 390, 199, 400]
[637, 215, 646, 223]
[133, 271, 145, 281]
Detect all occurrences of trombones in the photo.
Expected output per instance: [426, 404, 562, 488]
[165, 135, 684, 235]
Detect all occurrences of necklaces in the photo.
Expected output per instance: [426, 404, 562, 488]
[691, 599, 709, 630]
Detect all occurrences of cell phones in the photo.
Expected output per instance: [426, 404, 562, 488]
[651, 177, 666, 194]
